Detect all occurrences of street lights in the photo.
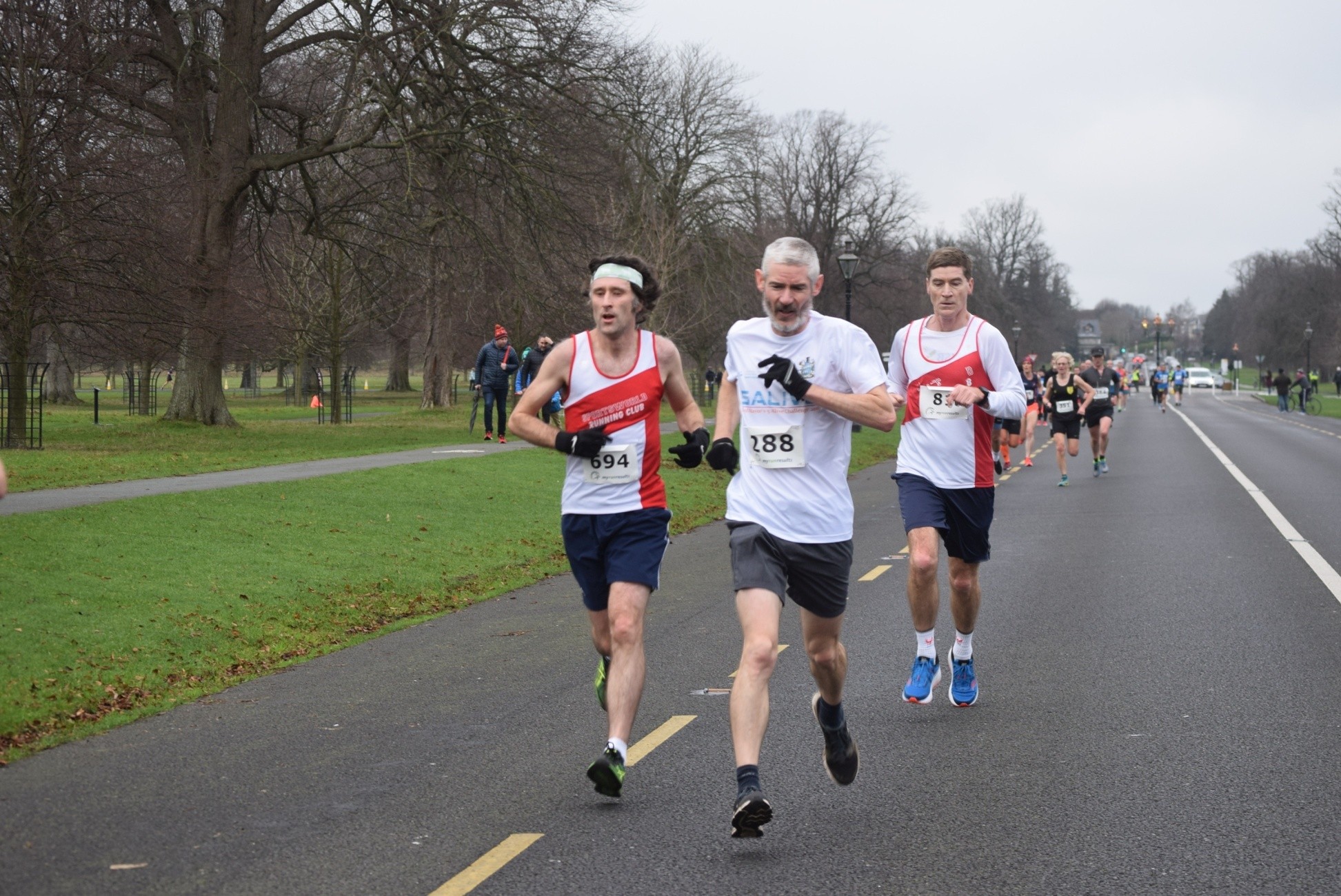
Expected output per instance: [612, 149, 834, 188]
[1141, 313, 1176, 367]
[1012, 320, 1022, 367]
[836, 239, 860, 322]
[1303, 321, 1314, 384]
[1232, 343, 1239, 390]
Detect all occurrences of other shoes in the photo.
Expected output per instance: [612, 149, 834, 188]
[1024, 456, 1033, 466]
[994, 459, 1002, 475]
[1019, 439, 1023, 444]
[1004, 461, 1012, 470]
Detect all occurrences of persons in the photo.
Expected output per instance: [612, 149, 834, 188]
[1289, 367, 1312, 416]
[1042, 352, 1094, 487]
[1037, 359, 1129, 438]
[514, 333, 562, 430]
[1081, 347, 1120, 477]
[508, 255, 704, 799]
[1150, 365, 1189, 413]
[1333, 366, 1341, 397]
[469, 367, 475, 390]
[1266, 370, 1272, 394]
[706, 366, 723, 399]
[1132, 366, 1140, 393]
[705, 237, 898, 837]
[991, 351, 1046, 475]
[474, 325, 519, 443]
[1309, 366, 1319, 394]
[1272, 369, 1292, 413]
[886, 247, 1026, 707]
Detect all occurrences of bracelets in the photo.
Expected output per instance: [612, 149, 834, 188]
[712, 438, 734, 447]
[695, 427, 709, 441]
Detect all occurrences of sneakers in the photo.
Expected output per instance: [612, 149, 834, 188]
[902, 654, 942, 704]
[484, 432, 493, 439]
[1098, 456, 1109, 473]
[587, 742, 625, 798]
[948, 646, 979, 707]
[594, 655, 612, 712]
[1058, 475, 1069, 486]
[731, 787, 773, 838]
[812, 691, 860, 787]
[1093, 459, 1100, 477]
[499, 434, 505, 443]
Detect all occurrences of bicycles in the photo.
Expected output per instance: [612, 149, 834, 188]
[1277, 387, 1322, 416]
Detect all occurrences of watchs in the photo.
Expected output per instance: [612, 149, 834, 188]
[977, 387, 989, 406]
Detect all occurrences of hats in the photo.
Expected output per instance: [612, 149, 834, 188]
[1091, 347, 1104, 356]
[495, 324, 508, 340]
[522, 347, 531, 359]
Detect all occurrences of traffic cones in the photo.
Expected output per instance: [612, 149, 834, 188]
[107, 379, 111, 390]
[364, 378, 368, 390]
[224, 379, 229, 390]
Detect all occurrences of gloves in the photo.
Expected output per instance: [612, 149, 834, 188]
[554, 425, 613, 458]
[758, 355, 812, 401]
[705, 437, 740, 476]
[668, 427, 709, 468]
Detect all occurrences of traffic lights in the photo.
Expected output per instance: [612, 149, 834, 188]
[1120, 344, 1126, 354]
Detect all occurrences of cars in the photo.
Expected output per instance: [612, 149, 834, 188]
[1113, 353, 1157, 388]
[1181, 367, 1215, 388]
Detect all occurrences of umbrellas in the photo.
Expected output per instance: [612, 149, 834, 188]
[470, 388, 480, 433]
